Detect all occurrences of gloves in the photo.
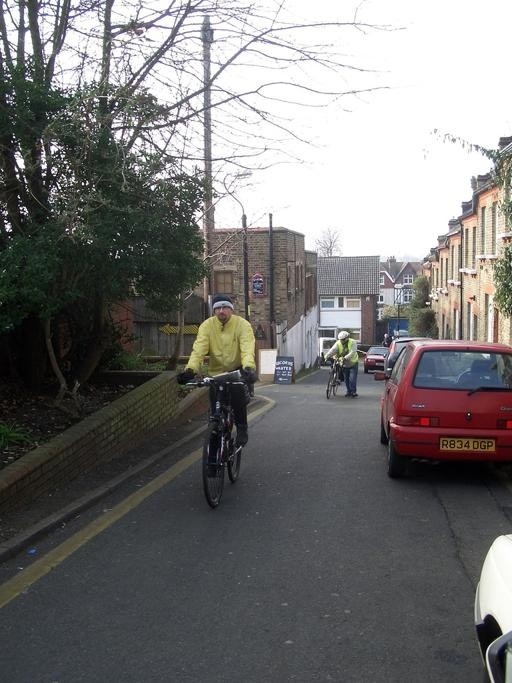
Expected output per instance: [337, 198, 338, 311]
[243, 367, 258, 385]
[176, 368, 198, 384]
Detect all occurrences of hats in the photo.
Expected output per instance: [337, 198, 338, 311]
[211, 295, 234, 313]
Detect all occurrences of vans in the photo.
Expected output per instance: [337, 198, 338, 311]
[319, 337, 337, 364]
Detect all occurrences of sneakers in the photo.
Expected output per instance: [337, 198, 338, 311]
[344, 392, 359, 399]
[236, 427, 249, 444]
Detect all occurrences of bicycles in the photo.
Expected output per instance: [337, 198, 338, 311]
[321, 350, 353, 400]
[182, 363, 252, 507]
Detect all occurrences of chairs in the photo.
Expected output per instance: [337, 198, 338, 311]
[463, 358, 498, 386]
[414, 357, 442, 388]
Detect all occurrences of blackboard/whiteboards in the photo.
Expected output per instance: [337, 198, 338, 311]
[274, 357, 294, 384]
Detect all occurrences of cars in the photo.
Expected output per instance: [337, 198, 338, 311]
[373, 339, 511, 479]
[382, 328, 408, 346]
[362, 346, 389, 373]
[472, 533, 511, 681]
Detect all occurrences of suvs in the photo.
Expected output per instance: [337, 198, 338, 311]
[383, 336, 439, 384]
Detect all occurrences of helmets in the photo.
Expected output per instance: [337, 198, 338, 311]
[338, 330, 351, 340]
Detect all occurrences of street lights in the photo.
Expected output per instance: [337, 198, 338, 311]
[222, 169, 252, 321]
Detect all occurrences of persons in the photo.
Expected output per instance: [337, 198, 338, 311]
[177, 293, 258, 477]
[383, 332, 390, 346]
[323, 330, 359, 399]
